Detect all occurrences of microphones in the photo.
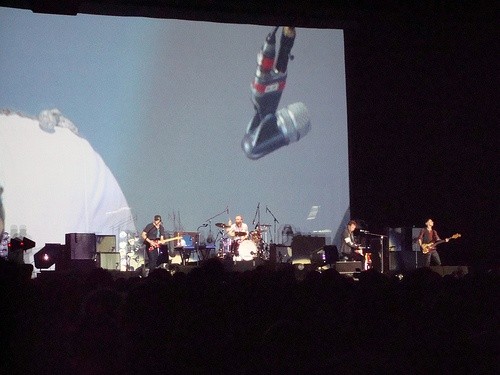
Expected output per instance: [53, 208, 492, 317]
[359, 229, 370, 233]
[239, 25, 311, 160]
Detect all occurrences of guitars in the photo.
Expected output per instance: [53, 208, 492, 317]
[148, 235, 182, 251]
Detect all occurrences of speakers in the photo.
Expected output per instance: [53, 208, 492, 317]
[315, 245, 338, 265]
[269, 244, 292, 263]
[382, 227, 402, 251]
[65, 233, 96, 260]
[383, 251, 418, 272]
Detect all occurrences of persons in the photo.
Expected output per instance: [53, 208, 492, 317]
[142, 215, 165, 273]
[0, 257, 500, 375]
[419, 217, 449, 266]
[341, 220, 362, 262]
[228, 216, 249, 256]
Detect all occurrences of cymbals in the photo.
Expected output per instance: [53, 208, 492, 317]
[215, 223, 231, 228]
[234, 231, 247, 236]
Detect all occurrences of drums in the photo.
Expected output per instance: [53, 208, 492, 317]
[249, 230, 262, 242]
[231, 239, 256, 261]
